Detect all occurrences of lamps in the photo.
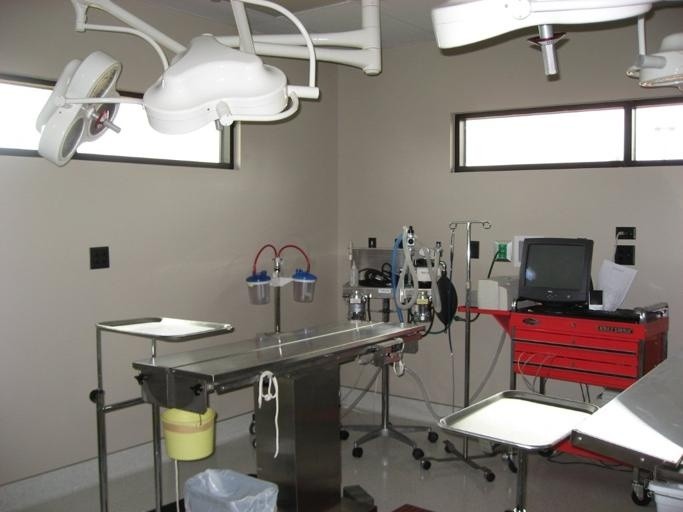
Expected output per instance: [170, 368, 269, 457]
[431, 0, 682, 88]
[29, 1, 384, 167]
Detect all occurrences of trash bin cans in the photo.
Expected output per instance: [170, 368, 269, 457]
[184, 469, 279, 512]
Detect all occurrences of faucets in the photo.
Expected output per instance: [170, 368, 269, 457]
[347, 241, 357, 288]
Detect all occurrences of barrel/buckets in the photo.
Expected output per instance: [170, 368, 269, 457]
[159, 407, 215, 461]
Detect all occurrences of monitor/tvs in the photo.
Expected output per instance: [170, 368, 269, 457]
[517, 236, 594, 315]
